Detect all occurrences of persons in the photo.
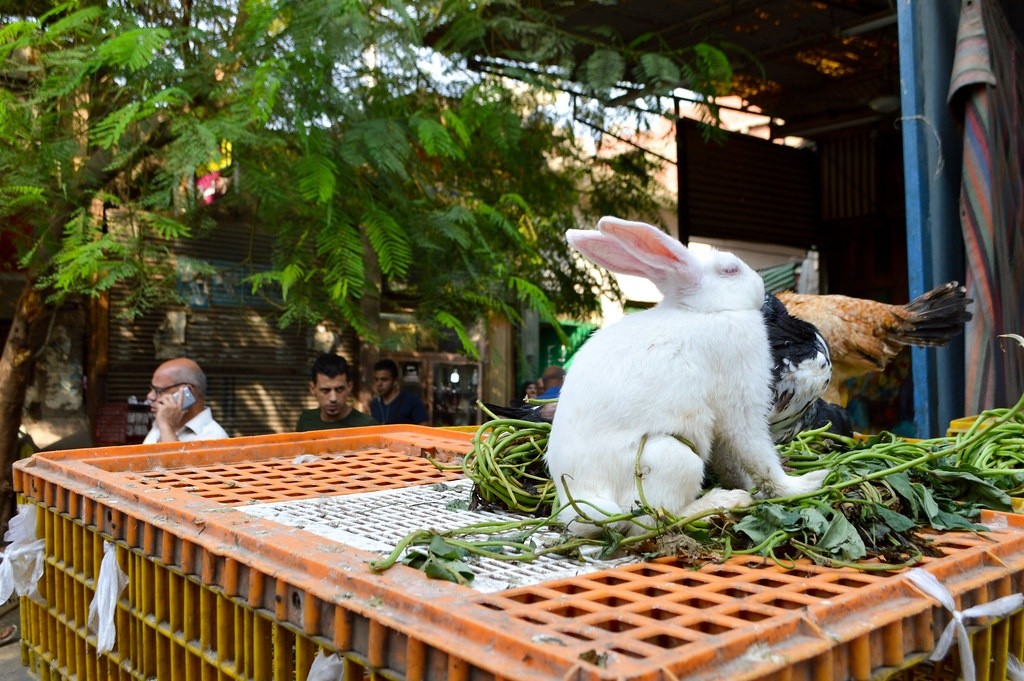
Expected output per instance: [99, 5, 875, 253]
[522, 367, 566, 399]
[296, 356, 430, 432]
[143, 357, 229, 443]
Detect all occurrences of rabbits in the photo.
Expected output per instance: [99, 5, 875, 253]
[544, 215, 830, 538]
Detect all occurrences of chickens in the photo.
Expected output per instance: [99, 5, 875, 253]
[485, 279, 974, 449]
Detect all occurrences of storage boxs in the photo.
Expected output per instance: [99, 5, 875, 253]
[12, 425, 1024, 681]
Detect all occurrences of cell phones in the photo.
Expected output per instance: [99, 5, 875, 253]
[173, 386, 196, 411]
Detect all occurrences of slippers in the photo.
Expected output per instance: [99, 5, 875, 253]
[0, 624, 18, 644]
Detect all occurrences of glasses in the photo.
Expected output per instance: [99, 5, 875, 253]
[148, 381, 195, 394]
